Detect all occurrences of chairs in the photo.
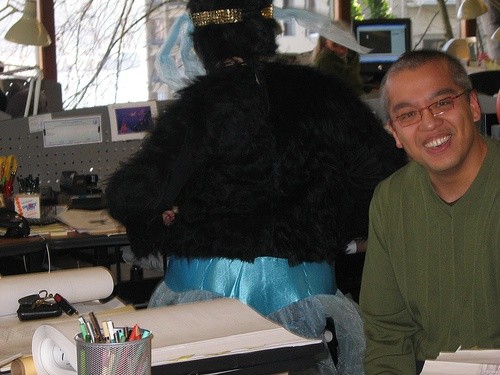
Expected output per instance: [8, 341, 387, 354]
[467, 69, 500, 137]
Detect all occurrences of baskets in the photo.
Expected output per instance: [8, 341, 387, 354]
[74, 327, 154, 375]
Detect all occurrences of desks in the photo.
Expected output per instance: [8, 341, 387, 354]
[0, 231, 131, 273]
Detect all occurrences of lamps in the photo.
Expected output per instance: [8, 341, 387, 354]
[441, 0, 500, 61]
[0, 0, 52, 118]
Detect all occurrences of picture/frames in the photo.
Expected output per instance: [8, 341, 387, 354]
[108, 100, 159, 143]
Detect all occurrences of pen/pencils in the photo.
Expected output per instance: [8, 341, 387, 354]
[78, 310, 151, 375]
[16, 174, 41, 194]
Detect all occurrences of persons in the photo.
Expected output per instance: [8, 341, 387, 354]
[314, 18, 379, 90]
[360, 47, 500, 375]
[104, 0, 410, 375]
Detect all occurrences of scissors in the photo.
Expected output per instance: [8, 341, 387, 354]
[0, 153, 16, 194]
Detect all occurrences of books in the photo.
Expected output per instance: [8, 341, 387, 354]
[420, 349, 500, 375]
[0, 206, 126, 237]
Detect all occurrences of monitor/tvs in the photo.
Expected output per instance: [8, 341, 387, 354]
[353, 17, 411, 82]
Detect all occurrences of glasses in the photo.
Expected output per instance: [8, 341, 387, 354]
[390, 91, 473, 128]
